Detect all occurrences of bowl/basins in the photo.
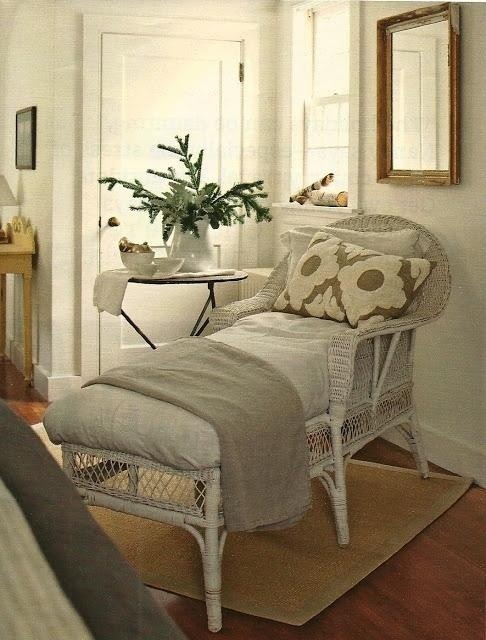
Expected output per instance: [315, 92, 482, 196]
[119, 251, 185, 277]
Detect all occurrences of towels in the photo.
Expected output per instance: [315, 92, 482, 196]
[81, 334, 314, 537]
[93, 268, 135, 316]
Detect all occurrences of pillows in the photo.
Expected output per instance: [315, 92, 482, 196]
[273, 226, 438, 330]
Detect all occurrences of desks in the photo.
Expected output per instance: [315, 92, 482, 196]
[0, 217, 39, 387]
[108, 266, 245, 352]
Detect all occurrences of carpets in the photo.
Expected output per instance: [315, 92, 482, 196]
[87, 458, 473, 627]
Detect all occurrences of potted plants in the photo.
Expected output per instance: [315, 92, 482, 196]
[97, 133, 271, 274]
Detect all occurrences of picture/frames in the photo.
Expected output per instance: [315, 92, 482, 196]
[15, 107, 36, 171]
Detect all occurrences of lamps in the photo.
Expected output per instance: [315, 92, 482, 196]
[0, 177, 19, 246]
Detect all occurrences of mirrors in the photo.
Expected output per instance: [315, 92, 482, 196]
[375, 3, 460, 188]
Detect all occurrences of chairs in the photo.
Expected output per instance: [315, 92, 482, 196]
[34, 213, 450, 636]
